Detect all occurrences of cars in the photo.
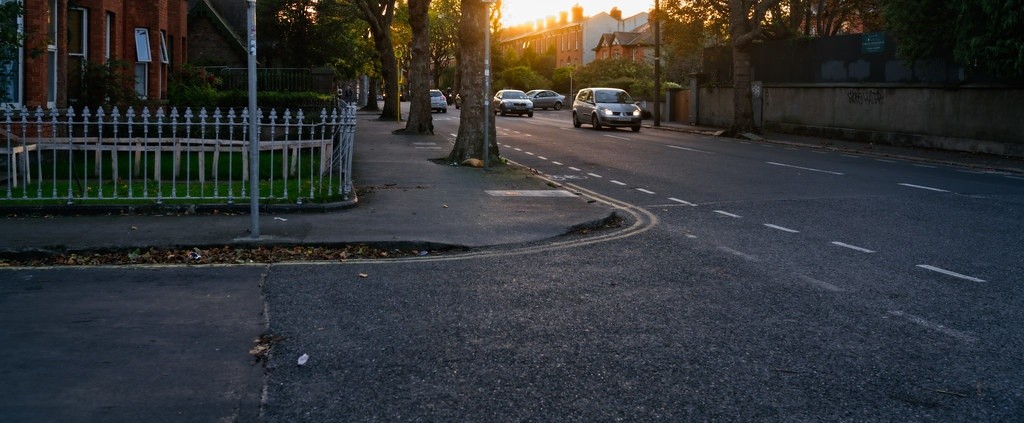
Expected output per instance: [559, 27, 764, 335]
[495, 88, 533, 117]
[430, 89, 447, 113]
[573, 87, 642, 132]
[524, 90, 567, 111]
